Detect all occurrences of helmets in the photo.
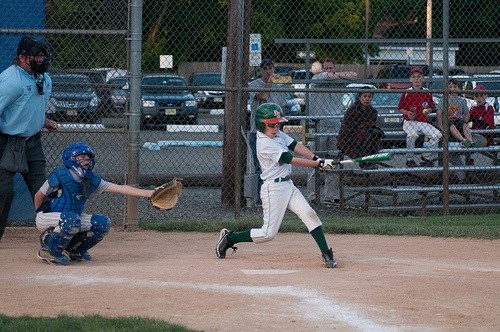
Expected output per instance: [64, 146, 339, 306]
[61, 142, 96, 178]
[254, 103, 288, 132]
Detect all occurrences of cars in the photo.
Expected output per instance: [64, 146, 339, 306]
[45, 74, 101, 122]
[247, 66, 314, 113]
[140, 74, 198, 128]
[104, 76, 130, 107]
[304, 63, 500, 143]
[186, 71, 225, 110]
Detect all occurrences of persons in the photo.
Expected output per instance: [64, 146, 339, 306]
[35, 141, 156, 265]
[0, 36, 59, 241]
[436, 78, 481, 148]
[465, 85, 494, 147]
[398, 67, 443, 167]
[248, 59, 286, 207]
[336, 86, 385, 169]
[308, 57, 357, 207]
[215, 103, 337, 268]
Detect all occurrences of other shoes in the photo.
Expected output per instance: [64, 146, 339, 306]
[421, 162, 434, 167]
[466, 159, 474, 165]
[463, 140, 482, 148]
[358, 163, 378, 169]
[406, 161, 418, 167]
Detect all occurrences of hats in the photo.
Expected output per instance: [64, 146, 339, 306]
[473, 85, 486, 90]
[410, 68, 424, 74]
[261, 58, 274, 65]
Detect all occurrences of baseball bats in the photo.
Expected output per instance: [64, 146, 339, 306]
[328, 152, 391, 166]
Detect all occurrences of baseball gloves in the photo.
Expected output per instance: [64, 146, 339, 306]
[150, 179, 184, 212]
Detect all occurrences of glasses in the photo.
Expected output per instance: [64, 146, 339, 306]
[34, 55, 47, 60]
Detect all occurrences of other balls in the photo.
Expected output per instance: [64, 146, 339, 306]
[423, 108, 428, 113]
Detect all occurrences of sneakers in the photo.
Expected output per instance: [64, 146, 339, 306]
[72, 252, 91, 262]
[36, 248, 70, 265]
[310, 199, 316, 205]
[215, 228, 238, 259]
[324, 199, 346, 206]
[322, 248, 337, 268]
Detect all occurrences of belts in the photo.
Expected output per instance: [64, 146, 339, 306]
[275, 175, 290, 183]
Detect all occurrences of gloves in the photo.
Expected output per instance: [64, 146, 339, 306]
[311, 155, 335, 170]
